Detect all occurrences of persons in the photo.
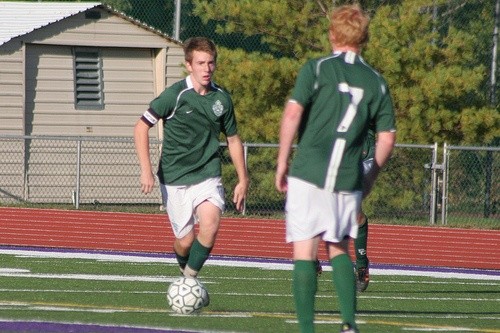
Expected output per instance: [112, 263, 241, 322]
[134, 37, 250, 279]
[274, 6, 396, 333]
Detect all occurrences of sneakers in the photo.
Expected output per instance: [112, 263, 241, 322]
[354, 261, 370, 296]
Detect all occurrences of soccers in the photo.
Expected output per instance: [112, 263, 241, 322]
[166, 276, 210, 315]
